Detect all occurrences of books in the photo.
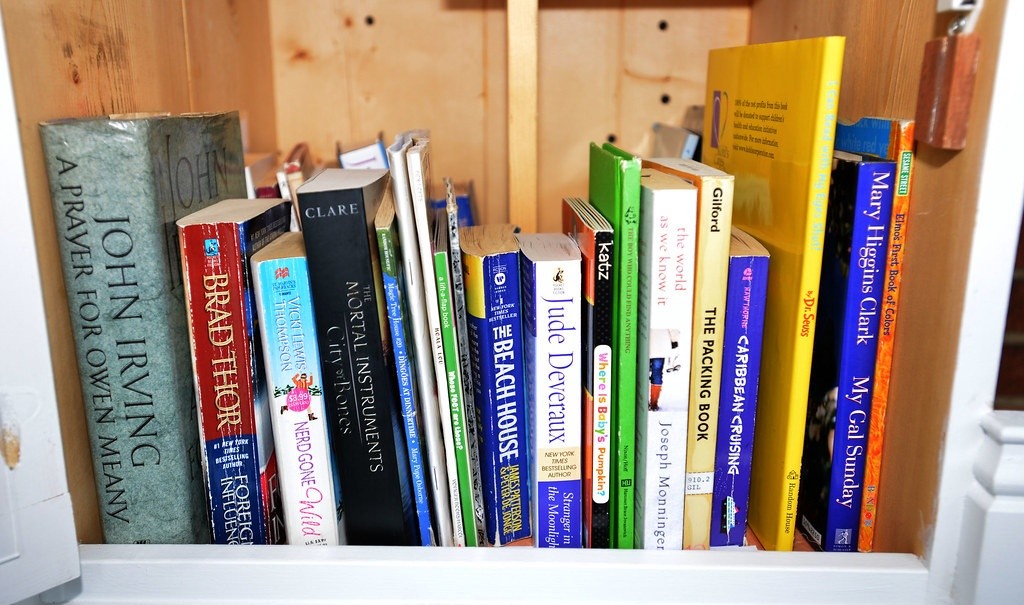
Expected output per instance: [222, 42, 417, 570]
[38, 107, 642, 549]
[797, 117, 917, 553]
[634, 34, 844, 553]
